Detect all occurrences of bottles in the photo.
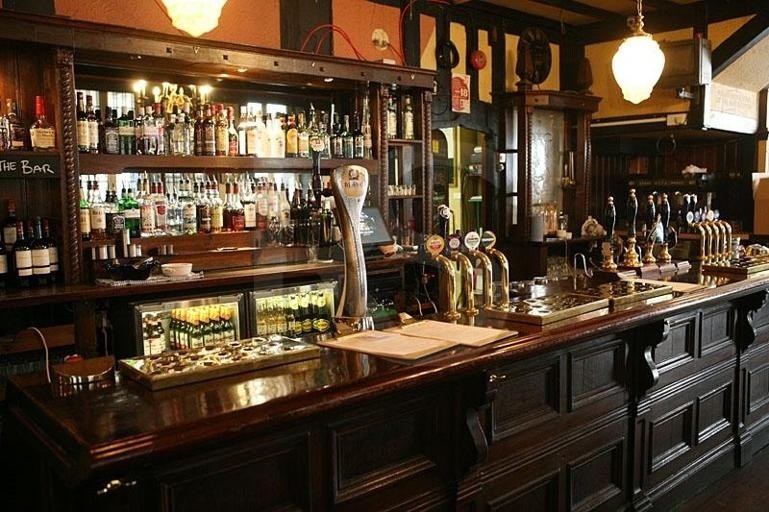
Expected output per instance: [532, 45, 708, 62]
[76, 177, 289, 259]
[141, 316, 166, 356]
[403, 96, 415, 139]
[389, 204, 417, 244]
[28, 94, 57, 152]
[1, 199, 60, 287]
[238, 94, 374, 161]
[76, 91, 237, 156]
[291, 188, 332, 246]
[606, 189, 698, 236]
[387, 95, 398, 140]
[169, 305, 236, 351]
[0, 99, 28, 152]
[253, 289, 327, 337]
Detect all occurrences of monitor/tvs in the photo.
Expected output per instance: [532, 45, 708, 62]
[330, 205, 395, 261]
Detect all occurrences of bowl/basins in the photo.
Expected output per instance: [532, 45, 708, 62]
[161, 263, 193, 276]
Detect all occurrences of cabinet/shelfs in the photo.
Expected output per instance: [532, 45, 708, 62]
[0, 15, 439, 382]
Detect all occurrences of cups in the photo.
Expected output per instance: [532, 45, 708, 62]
[269, 219, 320, 247]
